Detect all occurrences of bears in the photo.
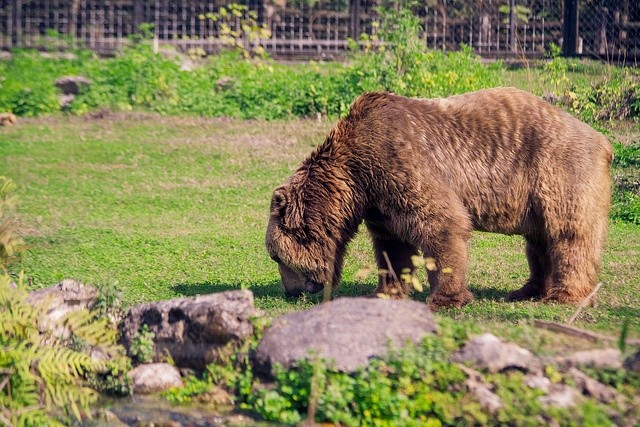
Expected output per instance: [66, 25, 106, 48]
[263, 85, 615, 321]
